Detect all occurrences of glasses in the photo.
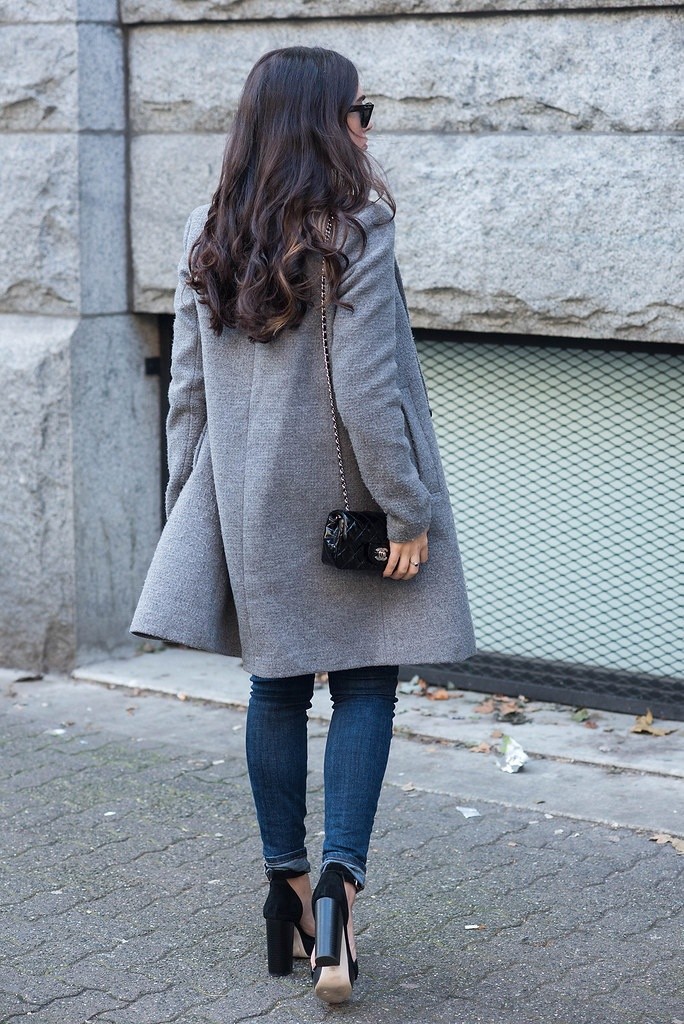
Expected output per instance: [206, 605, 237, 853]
[346, 102, 374, 128]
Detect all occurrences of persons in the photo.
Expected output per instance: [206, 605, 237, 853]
[128, 47, 477, 1003]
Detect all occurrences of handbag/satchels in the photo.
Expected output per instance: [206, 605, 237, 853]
[322, 509, 429, 574]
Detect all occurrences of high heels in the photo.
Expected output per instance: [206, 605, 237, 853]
[262, 868, 316, 978]
[310, 862, 362, 1004]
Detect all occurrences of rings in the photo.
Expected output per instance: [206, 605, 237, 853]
[411, 561, 419, 566]
